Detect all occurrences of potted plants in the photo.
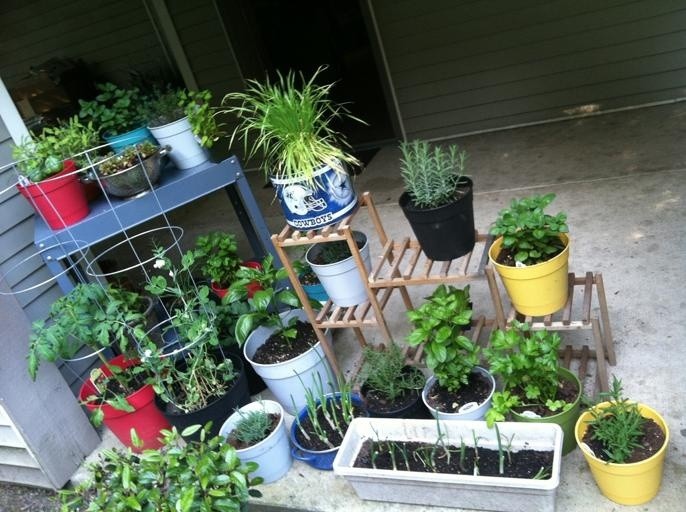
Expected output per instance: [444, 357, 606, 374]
[487, 192, 570, 317]
[397, 138, 475, 261]
[481, 319, 582, 456]
[405, 283, 496, 421]
[574, 373, 669, 505]
[350, 343, 427, 419]
[333, 417, 564, 512]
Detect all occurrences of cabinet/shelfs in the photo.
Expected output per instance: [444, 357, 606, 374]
[33, 145, 293, 376]
[270, 191, 616, 402]
